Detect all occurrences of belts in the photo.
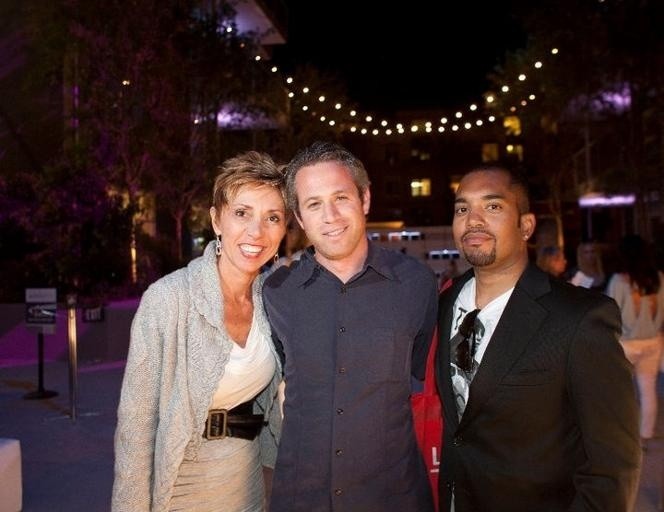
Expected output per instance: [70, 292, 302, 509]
[205, 400, 268, 440]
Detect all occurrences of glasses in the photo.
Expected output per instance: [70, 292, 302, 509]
[455, 308, 482, 373]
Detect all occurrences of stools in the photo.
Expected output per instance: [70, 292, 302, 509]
[0, 438, 22, 512]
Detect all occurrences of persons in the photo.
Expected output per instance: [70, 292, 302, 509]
[107, 148, 295, 511]
[260, 139, 444, 511]
[605, 231, 663, 442]
[430, 157, 644, 512]
[535, 230, 664, 293]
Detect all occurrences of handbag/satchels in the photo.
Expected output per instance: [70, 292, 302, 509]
[409, 390, 443, 512]
